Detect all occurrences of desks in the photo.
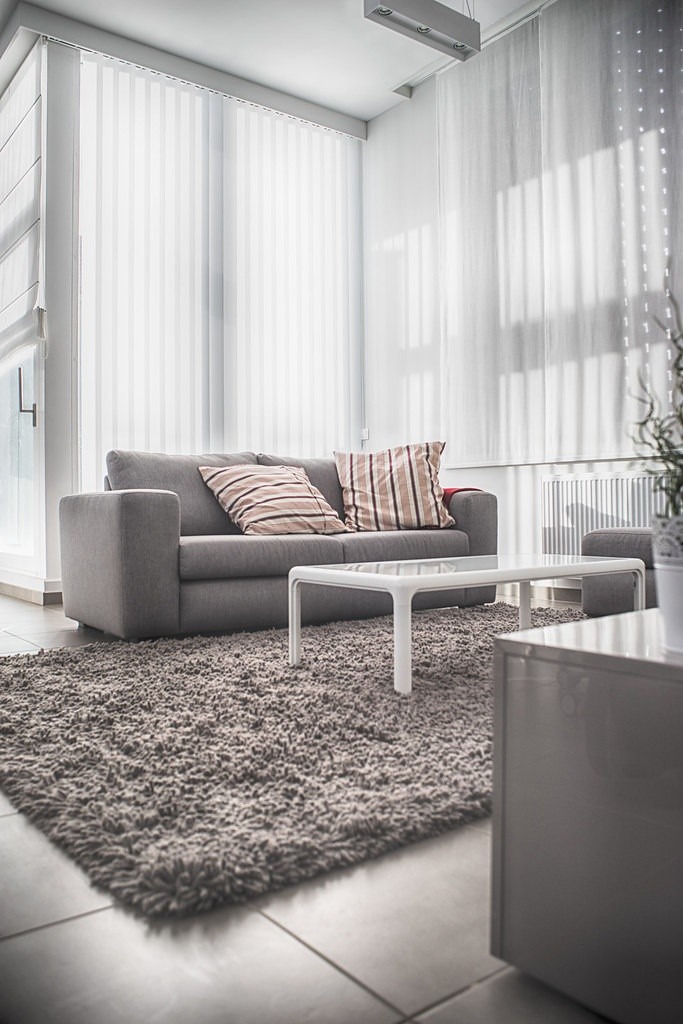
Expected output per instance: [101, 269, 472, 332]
[489, 606, 683, 1024]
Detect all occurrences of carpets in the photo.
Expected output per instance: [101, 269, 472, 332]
[0, 603, 662, 923]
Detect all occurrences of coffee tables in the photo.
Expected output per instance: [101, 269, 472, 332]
[288, 554, 645, 698]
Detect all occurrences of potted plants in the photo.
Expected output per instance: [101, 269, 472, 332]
[623, 253, 683, 656]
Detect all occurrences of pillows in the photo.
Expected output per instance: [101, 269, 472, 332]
[330, 439, 460, 534]
[198, 462, 359, 536]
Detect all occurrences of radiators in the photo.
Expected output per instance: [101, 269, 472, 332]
[541, 469, 683, 580]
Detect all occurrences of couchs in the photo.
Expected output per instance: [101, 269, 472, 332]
[58, 448, 499, 643]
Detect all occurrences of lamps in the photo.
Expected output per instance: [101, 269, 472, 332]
[363, 0, 481, 62]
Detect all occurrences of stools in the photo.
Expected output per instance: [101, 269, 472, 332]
[580, 526, 659, 617]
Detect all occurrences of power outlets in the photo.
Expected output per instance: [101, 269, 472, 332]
[361, 428, 368, 440]
